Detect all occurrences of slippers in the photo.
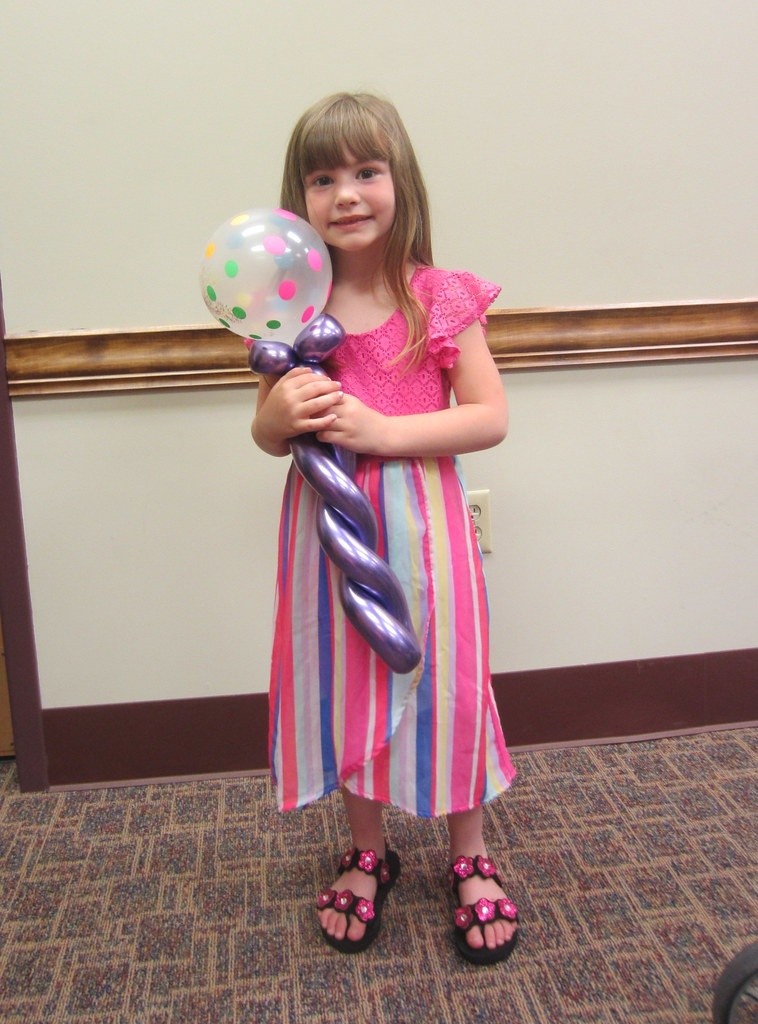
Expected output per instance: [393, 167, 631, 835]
[450, 856, 518, 963]
[316, 851, 399, 954]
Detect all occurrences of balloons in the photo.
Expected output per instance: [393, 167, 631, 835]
[200, 207, 421, 675]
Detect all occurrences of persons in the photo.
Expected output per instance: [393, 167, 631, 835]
[252, 93, 520, 965]
